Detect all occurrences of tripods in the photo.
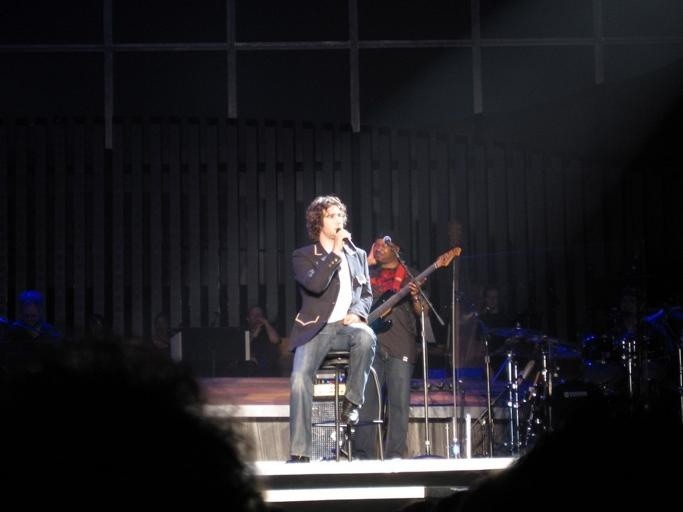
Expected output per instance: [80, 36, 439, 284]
[471, 341, 509, 456]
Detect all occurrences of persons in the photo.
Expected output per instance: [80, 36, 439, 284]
[287, 191, 378, 463]
[474, 286, 517, 353]
[614, 287, 673, 363]
[0, 328, 270, 510]
[348, 234, 432, 462]
[9, 302, 281, 380]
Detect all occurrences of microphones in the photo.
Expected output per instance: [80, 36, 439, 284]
[381, 233, 401, 267]
[335, 226, 360, 258]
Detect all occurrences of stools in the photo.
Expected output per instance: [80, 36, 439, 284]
[311, 350, 384, 461]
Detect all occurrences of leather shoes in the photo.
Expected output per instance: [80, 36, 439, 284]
[286, 455, 309, 464]
[342, 397, 360, 427]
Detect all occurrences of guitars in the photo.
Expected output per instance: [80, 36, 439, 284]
[368, 247, 461, 333]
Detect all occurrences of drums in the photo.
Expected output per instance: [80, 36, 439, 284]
[614, 334, 653, 362]
[580, 335, 616, 368]
